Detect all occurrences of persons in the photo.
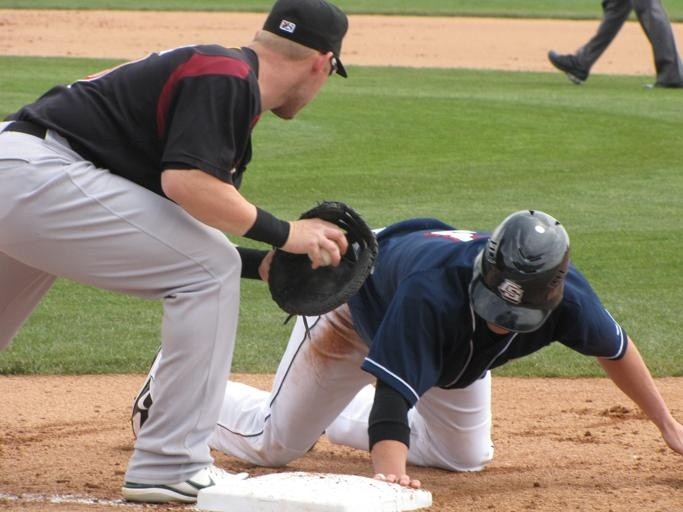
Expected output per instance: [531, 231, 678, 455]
[130, 210, 682, 489]
[548, 0, 683, 90]
[1, 2, 348, 503]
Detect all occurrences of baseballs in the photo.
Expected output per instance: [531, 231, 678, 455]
[307, 238, 340, 267]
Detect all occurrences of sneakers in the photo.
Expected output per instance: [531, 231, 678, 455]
[128, 345, 167, 445]
[548, 50, 588, 86]
[122, 465, 250, 507]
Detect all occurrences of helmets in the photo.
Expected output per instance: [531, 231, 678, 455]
[471, 208, 570, 336]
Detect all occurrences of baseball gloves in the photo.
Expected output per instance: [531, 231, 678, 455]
[266, 201, 379, 317]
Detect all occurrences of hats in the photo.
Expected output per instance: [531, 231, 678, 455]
[263, 1, 350, 79]
